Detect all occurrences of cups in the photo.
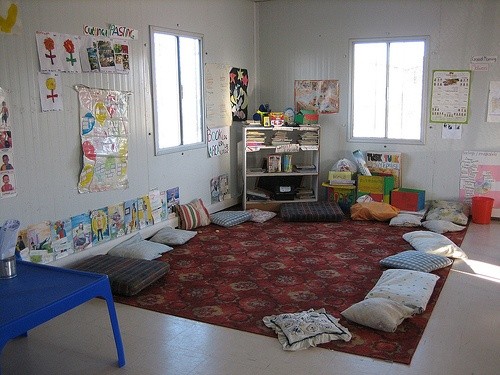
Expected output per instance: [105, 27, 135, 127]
[0, 255, 18, 279]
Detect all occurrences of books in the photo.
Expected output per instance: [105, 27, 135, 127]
[296, 131, 319, 145]
[246, 131, 266, 146]
[269, 130, 293, 146]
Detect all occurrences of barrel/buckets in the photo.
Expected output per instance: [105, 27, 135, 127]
[472, 197, 495, 224]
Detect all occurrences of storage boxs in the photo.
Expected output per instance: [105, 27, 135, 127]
[390, 186, 426, 212]
[357, 172, 395, 203]
[294, 109, 319, 125]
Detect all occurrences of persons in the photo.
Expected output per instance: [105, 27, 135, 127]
[111, 211, 121, 229]
[0, 101, 9, 127]
[168, 191, 177, 203]
[94, 213, 106, 241]
[0, 134, 10, 149]
[128, 201, 137, 233]
[0, 174, 14, 192]
[56, 224, 68, 254]
[30, 235, 50, 252]
[73, 222, 86, 246]
[15, 235, 25, 258]
[0, 154, 14, 171]
[141, 200, 149, 224]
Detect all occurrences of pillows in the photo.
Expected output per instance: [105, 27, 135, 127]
[422, 221, 467, 234]
[249, 209, 276, 223]
[378, 250, 454, 274]
[174, 198, 211, 230]
[262, 307, 352, 352]
[339, 297, 421, 334]
[389, 213, 424, 227]
[426, 199, 471, 225]
[364, 269, 441, 314]
[209, 211, 251, 228]
[149, 226, 198, 245]
[108, 232, 174, 260]
[349, 202, 402, 223]
[402, 230, 469, 259]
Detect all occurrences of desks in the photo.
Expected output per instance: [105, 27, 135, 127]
[0, 259, 125, 375]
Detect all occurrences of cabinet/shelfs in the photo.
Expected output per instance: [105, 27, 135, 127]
[241, 126, 320, 210]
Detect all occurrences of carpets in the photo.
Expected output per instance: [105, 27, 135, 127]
[98, 215, 468, 365]
